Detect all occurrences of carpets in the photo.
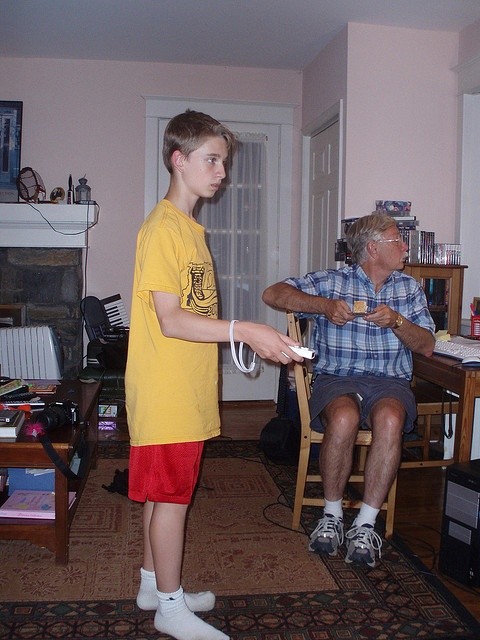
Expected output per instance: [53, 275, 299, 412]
[0, 439, 479, 639]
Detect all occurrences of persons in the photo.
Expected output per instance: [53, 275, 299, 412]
[125, 110, 304, 640]
[262, 214, 436, 573]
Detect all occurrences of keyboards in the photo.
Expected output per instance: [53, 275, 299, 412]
[433, 340, 480, 361]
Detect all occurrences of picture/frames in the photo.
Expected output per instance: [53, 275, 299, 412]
[0, 100, 23, 204]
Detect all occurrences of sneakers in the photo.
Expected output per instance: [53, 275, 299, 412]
[308, 513, 345, 556]
[344, 518, 383, 572]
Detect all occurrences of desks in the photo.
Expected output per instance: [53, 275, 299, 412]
[0, 380, 104, 566]
[409, 334, 480, 464]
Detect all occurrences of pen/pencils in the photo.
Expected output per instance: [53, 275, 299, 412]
[470, 300, 480, 316]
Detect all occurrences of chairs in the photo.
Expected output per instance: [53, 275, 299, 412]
[286, 310, 399, 540]
[78, 339, 127, 430]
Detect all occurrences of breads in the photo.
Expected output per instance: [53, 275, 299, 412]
[354, 301, 366, 314]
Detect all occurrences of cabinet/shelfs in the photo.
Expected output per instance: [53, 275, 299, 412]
[401, 262, 468, 335]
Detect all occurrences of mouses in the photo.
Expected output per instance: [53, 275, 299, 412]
[461, 356, 480, 367]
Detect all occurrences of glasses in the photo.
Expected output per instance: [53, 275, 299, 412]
[380, 233, 403, 244]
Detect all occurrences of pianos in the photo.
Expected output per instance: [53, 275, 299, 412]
[100, 293, 129, 329]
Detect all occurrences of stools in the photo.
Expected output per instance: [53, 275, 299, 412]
[400, 383, 460, 469]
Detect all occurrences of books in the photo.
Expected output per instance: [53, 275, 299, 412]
[0, 489, 77, 519]
[418, 231, 435, 264]
[434, 243, 461, 265]
[1, 411, 26, 438]
[399, 231, 419, 263]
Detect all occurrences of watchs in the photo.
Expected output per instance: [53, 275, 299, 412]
[392, 311, 403, 329]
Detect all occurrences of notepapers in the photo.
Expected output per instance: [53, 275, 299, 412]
[435, 329, 450, 340]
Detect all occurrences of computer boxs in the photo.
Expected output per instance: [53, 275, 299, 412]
[436, 457, 478, 590]
